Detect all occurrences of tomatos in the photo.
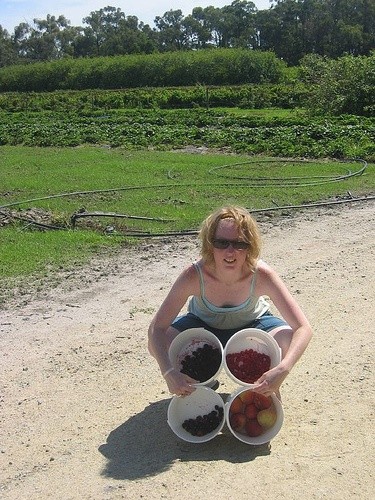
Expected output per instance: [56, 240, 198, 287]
[226, 348, 271, 384]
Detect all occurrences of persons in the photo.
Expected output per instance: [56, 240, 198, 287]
[147, 205, 315, 404]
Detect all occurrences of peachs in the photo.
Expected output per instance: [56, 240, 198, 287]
[230, 391, 276, 438]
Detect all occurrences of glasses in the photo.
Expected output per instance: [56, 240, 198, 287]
[211, 237, 251, 251]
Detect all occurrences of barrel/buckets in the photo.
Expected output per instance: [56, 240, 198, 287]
[170, 327, 224, 388]
[167, 385, 225, 444]
[223, 327, 282, 387]
[223, 388, 284, 445]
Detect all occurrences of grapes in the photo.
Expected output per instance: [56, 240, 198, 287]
[179, 343, 222, 382]
[181, 405, 224, 436]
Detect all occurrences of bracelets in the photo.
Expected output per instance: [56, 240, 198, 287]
[162, 368, 174, 378]
